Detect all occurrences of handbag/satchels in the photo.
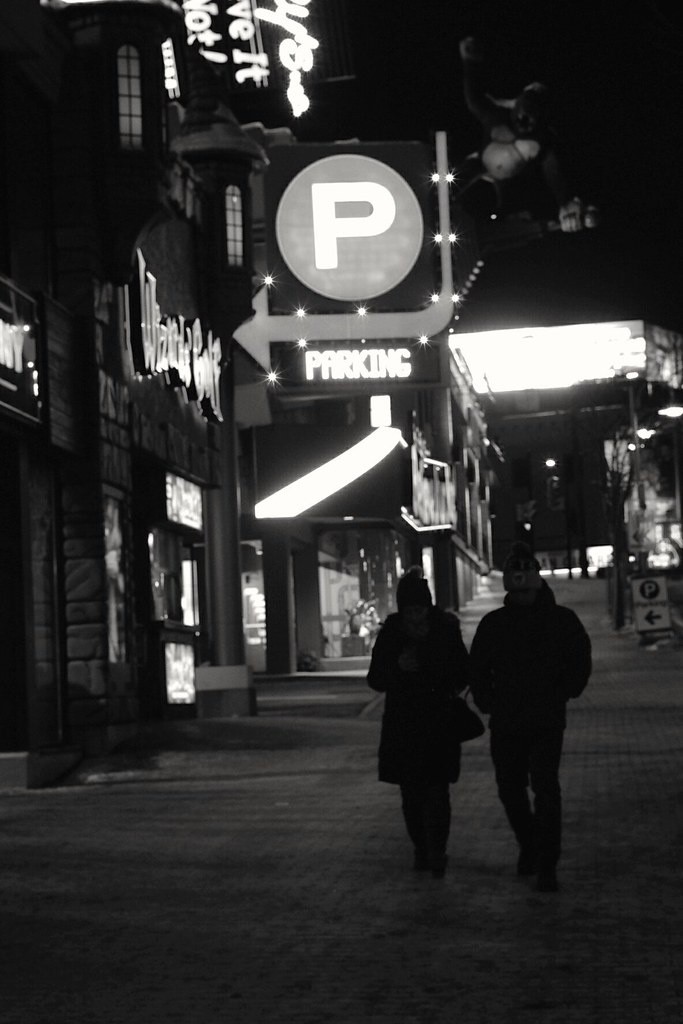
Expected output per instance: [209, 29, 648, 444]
[447, 693, 484, 742]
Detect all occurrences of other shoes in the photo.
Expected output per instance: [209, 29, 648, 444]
[414, 851, 447, 877]
[517, 853, 559, 894]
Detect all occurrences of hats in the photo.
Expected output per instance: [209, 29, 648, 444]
[396, 566, 433, 609]
[504, 542, 540, 591]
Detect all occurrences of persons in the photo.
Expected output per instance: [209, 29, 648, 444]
[366, 542, 593, 893]
